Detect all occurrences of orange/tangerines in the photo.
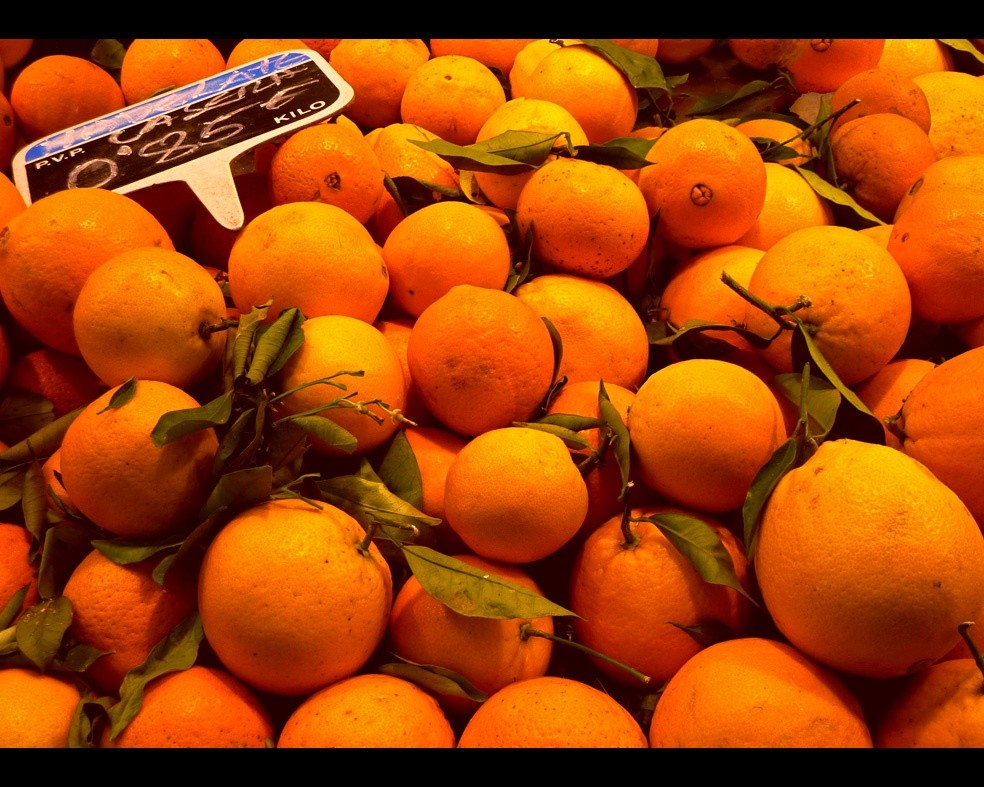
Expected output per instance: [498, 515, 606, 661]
[0, 39, 984, 747]
